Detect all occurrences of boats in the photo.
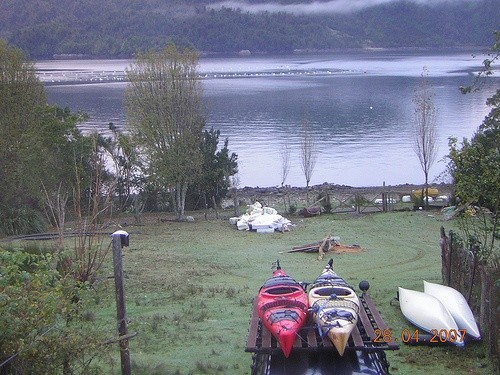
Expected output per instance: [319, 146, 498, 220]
[422, 278, 483, 343]
[396, 286, 464, 348]
[256, 261, 310, 360]
[306, 261, 360, 358]
